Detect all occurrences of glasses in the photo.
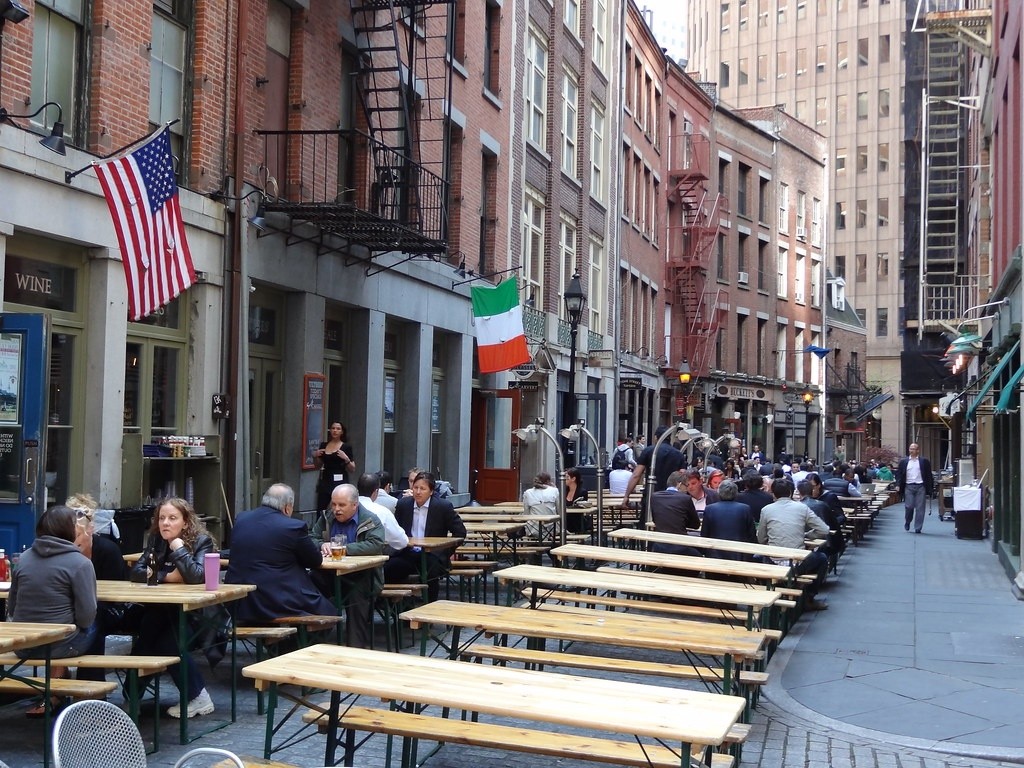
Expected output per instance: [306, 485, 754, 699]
[75, 510, 91, 522]
[679, 481, 688, 488]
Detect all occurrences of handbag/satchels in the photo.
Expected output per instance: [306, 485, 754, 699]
[577, 501, 592, 509]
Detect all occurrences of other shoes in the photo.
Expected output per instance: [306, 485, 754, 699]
[915, 529, 921, 533]
[905, 523, 909, 530]
[802, 599, 828, 610]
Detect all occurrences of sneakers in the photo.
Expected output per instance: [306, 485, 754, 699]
[121, 699, 140, 714]
[166, 686, 215, 719]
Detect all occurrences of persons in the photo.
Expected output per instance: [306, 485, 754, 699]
[9, 506, 119, 738]
[374, 470, 398, 514]
[622, 425, 683, 530]
[312, 420, 355, 522]
[222, 483, 339, 656]
[522, 471, 593, 568]
[24, 493, 131, 717]
[355, 473, 414, 583]
[896, 444, 932, 533]
[658, 445, 896, 610]
[608, 434, 646, 494]
[121, 497, 233, 718]
[309, 483, 386, 648]
[397, 467, 440, 500]
[394, 472, 466, 604]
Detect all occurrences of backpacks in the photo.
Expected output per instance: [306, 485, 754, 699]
[612, 447, 630, 470]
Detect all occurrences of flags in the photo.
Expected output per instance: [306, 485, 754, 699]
[471, 274, 530, 374]
[91, 124, 196, 322]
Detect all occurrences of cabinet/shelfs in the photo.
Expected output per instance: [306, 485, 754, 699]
[119, 433, 224, 552]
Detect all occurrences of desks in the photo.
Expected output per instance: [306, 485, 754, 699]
[405, 535, 464, 641]
[0, 580, 258, 747]
[609, 528, 811, 613]
[0, 621, 77, 767]
[492, 563, 782, 723]
[122, 553, 389, 651]
[398, 600, 767, 767]
[242, 644, 746, 768]
[547, 542, 792, 654]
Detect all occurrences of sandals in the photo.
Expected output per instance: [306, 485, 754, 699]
[52, 666, 71, 679]
[25, 695, 66, 717]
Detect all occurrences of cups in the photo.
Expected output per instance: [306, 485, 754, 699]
[335, 533, 347, 561]
[205, 553, 220, 591]
[330, 537, 342, 562]
[156, 477, 194, 513]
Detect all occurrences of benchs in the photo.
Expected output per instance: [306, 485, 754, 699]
[0, 488, 900, 768]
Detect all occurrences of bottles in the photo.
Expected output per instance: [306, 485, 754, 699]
[0, 544, 26, 581]
[151, 435, 205, 457]
[146, 548, 157, 586]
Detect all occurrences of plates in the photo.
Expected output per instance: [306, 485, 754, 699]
[143, 445, 173, 457]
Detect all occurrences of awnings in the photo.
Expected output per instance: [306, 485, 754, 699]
[946, 340, 1024, 425]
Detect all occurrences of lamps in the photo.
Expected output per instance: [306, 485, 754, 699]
[0, 102, 67, 157]
[946, 297, 1011, 354]
[518, 285, 535, 308]
[422, 251, 466, 279]
[0, 0, 30, 24]
[211, 190, 266, 232]
[512, 418, 742, 568]
[627, 347, 668, 364]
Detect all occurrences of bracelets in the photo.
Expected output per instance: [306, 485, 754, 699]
[346, 461, 350, 465]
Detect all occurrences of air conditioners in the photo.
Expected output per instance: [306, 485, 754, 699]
[797, 227, 807, 237]
[737, 271, 749, 284]
